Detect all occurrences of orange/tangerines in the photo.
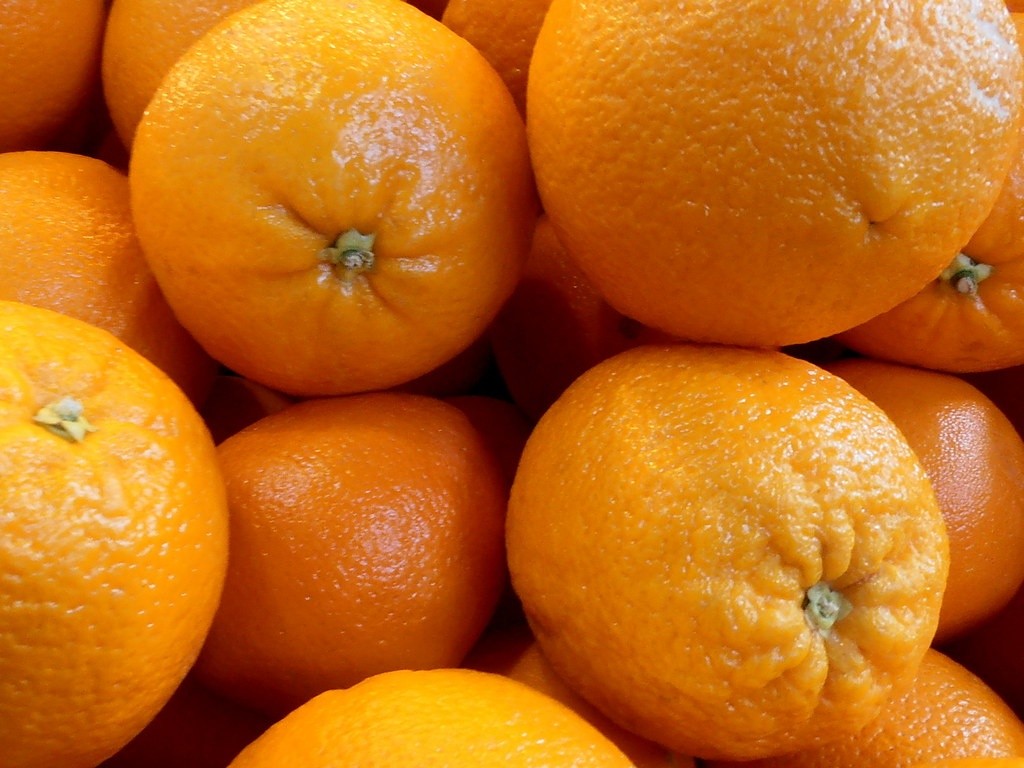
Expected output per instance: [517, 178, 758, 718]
[1, 1, 1024, 768]
